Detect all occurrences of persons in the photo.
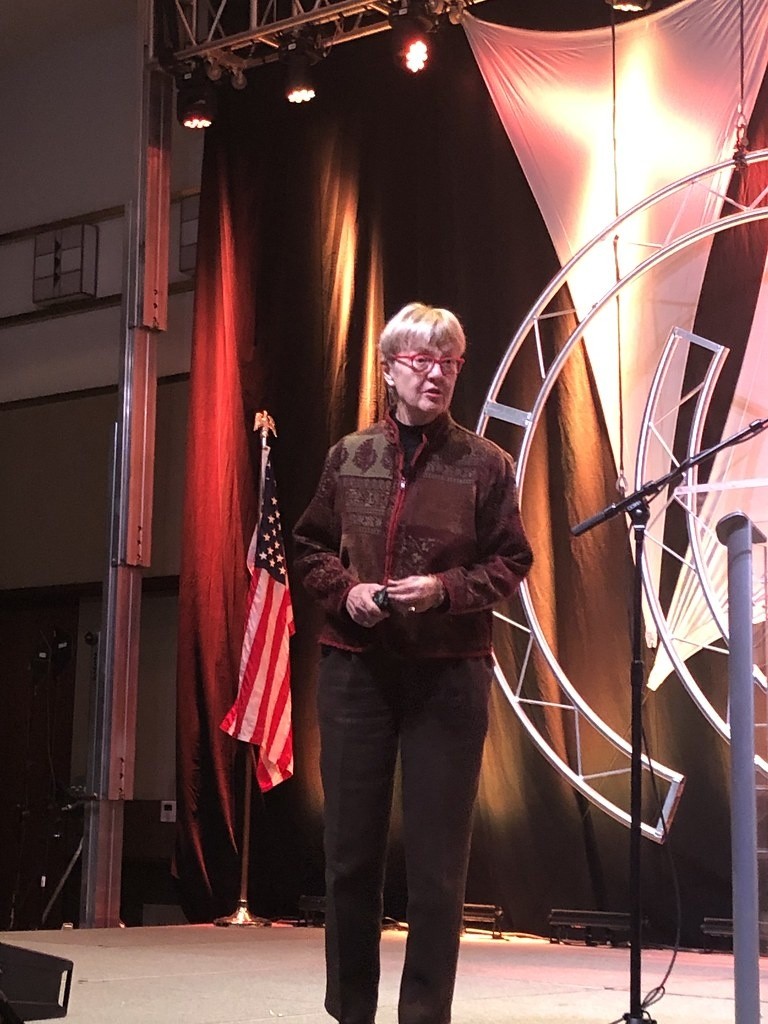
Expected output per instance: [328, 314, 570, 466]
[289, 302, 534, 1021]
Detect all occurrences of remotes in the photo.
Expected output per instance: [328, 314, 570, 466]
[373, 585, 389, 610]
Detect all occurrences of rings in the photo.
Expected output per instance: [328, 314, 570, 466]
[407, 606, 416, 614]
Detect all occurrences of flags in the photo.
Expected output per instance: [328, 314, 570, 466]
[219, 425, 294, 796]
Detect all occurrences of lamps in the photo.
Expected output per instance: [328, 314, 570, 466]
[701, 916, 768, 957]
[282, 40, 316, 104]
[548, 909, 650, 950]
[462, 902, 504, 940]
[176, 71, 217, 129]
[388, 15, 432, 77]
[297, 895, 327, 928]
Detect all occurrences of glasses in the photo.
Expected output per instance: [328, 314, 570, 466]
[395, 353, 465, 375]
